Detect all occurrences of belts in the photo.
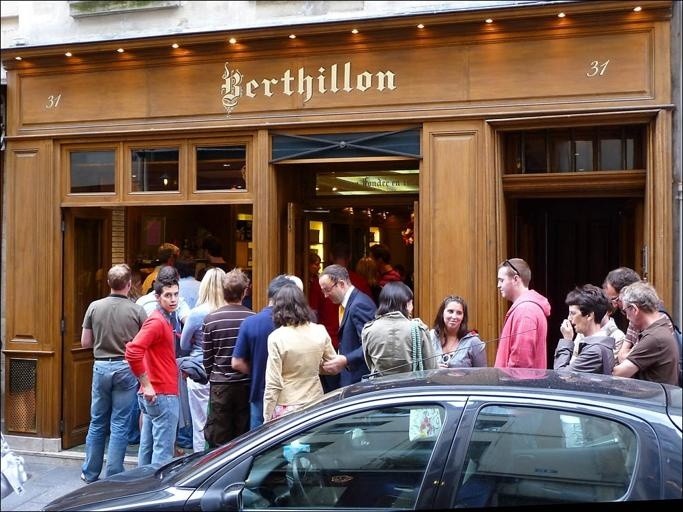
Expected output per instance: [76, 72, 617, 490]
[95, 357, 125, 361]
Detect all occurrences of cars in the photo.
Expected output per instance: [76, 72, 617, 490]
[45, 366, 682, 512]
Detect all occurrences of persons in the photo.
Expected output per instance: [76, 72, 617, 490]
[369, 368, 667, 399]
[493, 257, 552, 370]
[308, 243, 401, 349]
[555, 266, 682, 388]
[361, 281, 440, 375]
[127, 242, 338, 468]
[429, 295, 487, 367]
[80, 263, 147, 483]
[318, 264, 378, 385]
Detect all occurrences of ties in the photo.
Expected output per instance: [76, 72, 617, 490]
[339, 305, 344, 327]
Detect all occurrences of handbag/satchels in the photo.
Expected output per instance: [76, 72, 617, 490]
[409, 409, 442, 442]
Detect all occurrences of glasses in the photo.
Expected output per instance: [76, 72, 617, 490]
[443, 354, 451, 365]
[503, 259, 520, 275]
[325, 282, 337, 293]
[621, 306, 632, 316]
